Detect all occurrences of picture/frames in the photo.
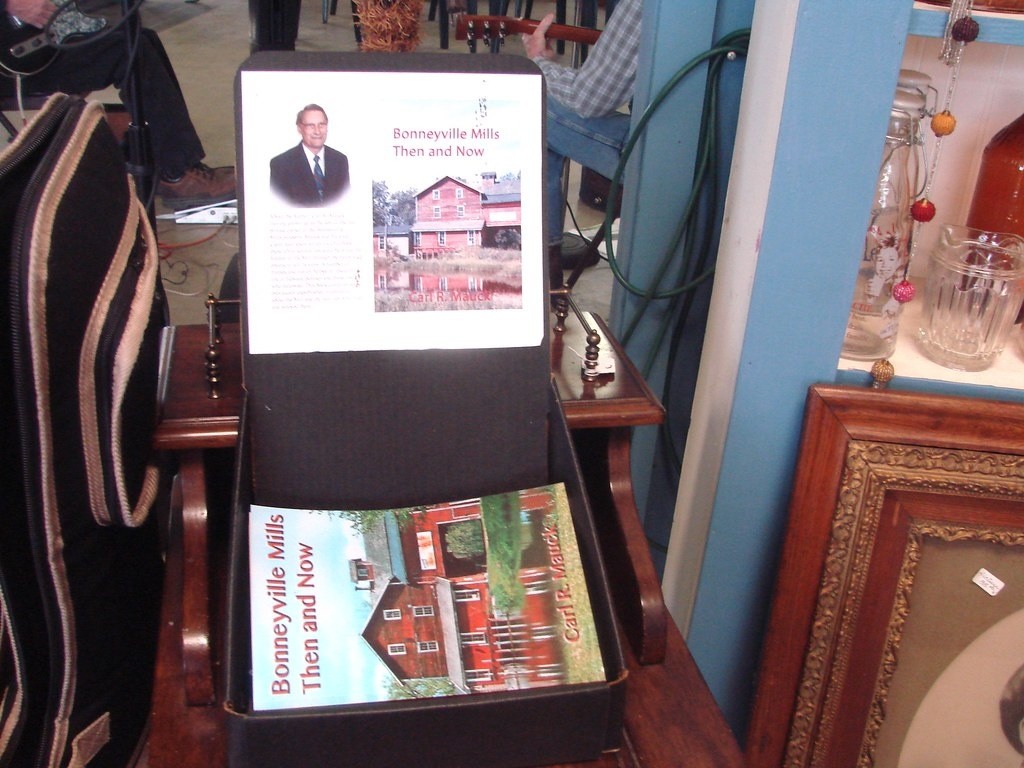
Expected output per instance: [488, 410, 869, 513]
[746, 380, 1024, 767]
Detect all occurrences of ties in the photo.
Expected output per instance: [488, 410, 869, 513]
[313, 155, 325, 204]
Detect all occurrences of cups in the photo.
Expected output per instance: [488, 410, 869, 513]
[914, 223, 1024, 373]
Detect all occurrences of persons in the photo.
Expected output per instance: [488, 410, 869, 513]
[520, 0, 642, 240]
[0, 0, 239, 210]
[269, 104, 349, 207]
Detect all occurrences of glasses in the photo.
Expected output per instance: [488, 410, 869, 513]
[300, 122, 328, 130]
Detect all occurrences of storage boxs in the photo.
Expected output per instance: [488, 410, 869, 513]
[217, 382, 630, 768]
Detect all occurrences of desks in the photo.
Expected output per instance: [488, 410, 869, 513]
[148, 326, 744, 768]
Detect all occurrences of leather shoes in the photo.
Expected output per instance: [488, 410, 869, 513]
[160, 161, 238, 209]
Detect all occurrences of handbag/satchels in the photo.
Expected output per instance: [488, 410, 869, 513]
[0, 91, 172, 768]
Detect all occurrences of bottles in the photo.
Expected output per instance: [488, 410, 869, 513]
[966, 113, 1024, 325]
[840, 68, 932, 362]
